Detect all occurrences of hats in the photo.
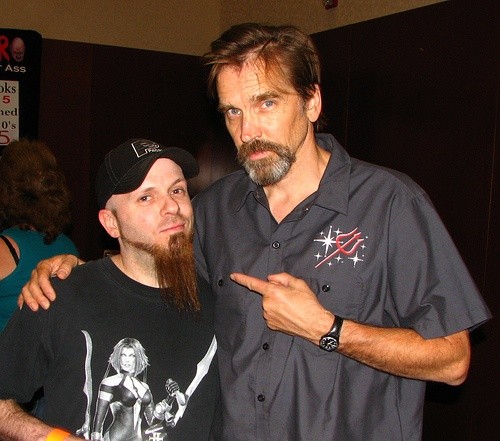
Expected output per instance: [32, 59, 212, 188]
[94, 140, 200, 210]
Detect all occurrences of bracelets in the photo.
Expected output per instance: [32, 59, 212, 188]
[44, 428, 70, 441]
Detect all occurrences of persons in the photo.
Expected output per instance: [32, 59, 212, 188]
[15, 21, 494, 440]
[0, 137, 221, 441]
[0, 140, 79, 330]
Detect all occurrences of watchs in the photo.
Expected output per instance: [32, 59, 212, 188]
[318, 314, 346, 354]
